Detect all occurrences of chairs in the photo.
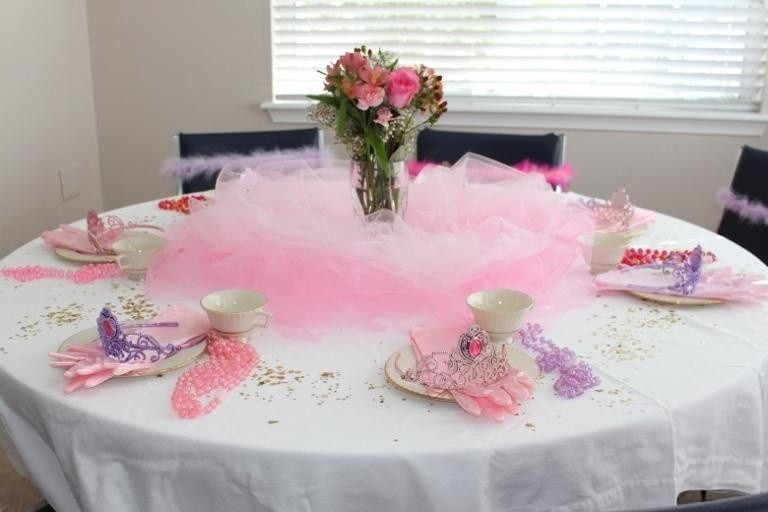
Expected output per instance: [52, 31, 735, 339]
[414, 127, 566, 194]
[714, 143, 768, 268]
[178, 127, 325, 195]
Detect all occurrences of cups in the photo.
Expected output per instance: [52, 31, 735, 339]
[582, 231, 628, 274]
[466, 289, 534, 339]
[202, 291, 274, 332]
[115, 236, 170, 271]
[351, 159, 409, 221]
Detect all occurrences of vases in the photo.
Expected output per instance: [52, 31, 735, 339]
[346, 160, 410, 226]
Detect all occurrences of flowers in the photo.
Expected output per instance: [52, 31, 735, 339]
[305, 38, 452, 214]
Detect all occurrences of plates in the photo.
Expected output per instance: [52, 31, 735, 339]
[385, 341, 541, 404]
[56, 225, 166, 263]
[58, 317, 207, 377]
[620, 277, 727, 305]
